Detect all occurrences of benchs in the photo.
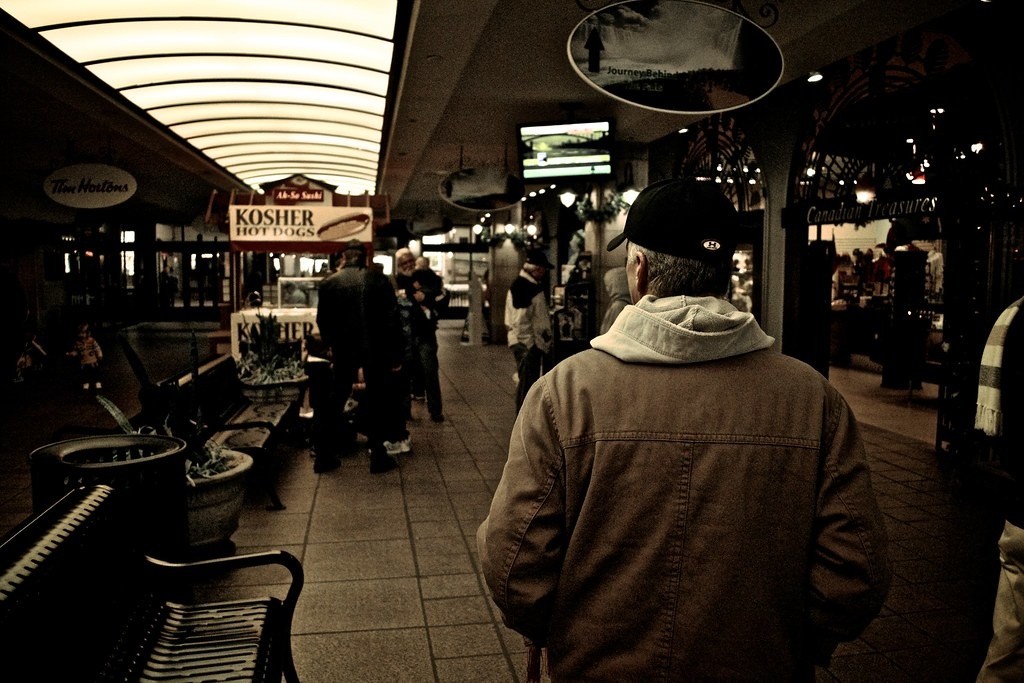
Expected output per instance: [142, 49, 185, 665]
[168, 358, 311, 510]
[0, 474, 304, 683]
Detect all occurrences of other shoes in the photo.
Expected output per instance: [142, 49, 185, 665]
[370, 456, 399, 472]
[82, 383, 89, 390]
[96, 382, 102, 389]
[410, 394, 425, 401]
[314, 456, 340, 473]
[431, 412, 444, 422]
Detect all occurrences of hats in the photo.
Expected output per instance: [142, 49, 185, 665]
[606, 177, 740, 261]
[525, 251, 555, 269]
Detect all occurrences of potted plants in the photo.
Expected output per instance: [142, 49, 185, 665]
[234, 309, 309, 402]
[114, 327, 254, 560]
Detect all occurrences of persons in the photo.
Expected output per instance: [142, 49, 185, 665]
[390, 246, 444, 423]
[318, 241, 406, 474]
[974, 300, 1024, 683]
[504, 251, 554, 420]
[65, 322, 104, 390]
[304, 335, 348, 474]
[477, 178, 891, 683]
[460, 269, 491, 343]
[600, 267, 632, 335]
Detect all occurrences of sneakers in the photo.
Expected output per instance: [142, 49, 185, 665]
[368, 435, 412, 454]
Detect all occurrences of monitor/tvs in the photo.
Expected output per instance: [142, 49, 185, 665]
[515, 119, 615, 186]
